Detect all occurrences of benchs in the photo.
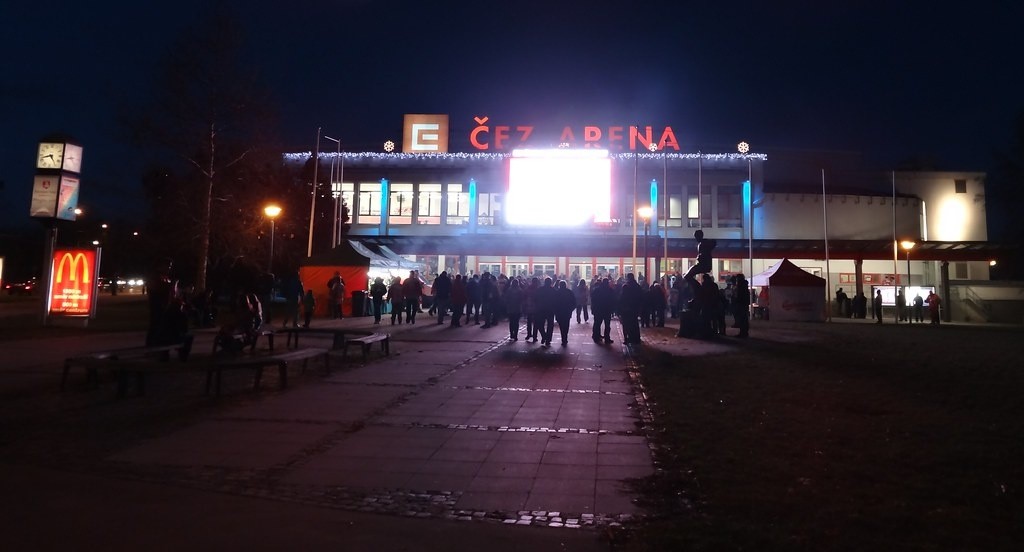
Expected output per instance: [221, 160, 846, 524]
[65, 326, 391, 407]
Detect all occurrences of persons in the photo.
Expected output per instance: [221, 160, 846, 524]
[836, 287, 867, 319]
[590, 271, 683, 346]
[428, 271, 589, 349]
[326, 271, 347, 320]
[282, 271, 315, 329]
[255, 263, 275, 325]
[371, 270, 422, 326]
[683, 230, 717, 304]
[875, 289, 882, 325]
[701, 273, 751, 338]
[897, 290, 940, 327]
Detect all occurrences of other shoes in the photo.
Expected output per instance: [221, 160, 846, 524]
[428, 311, 432, 316]
[533, 339, 537, 343]
[542, 335, 546, 344]
[434, 309, 437, 313]
[605, 340, 613, 344]
[375, 317, 498, 328]
[525, 335, 532, 340]
[418, 310, 422, 313]
[545, 343, 551, 348]
